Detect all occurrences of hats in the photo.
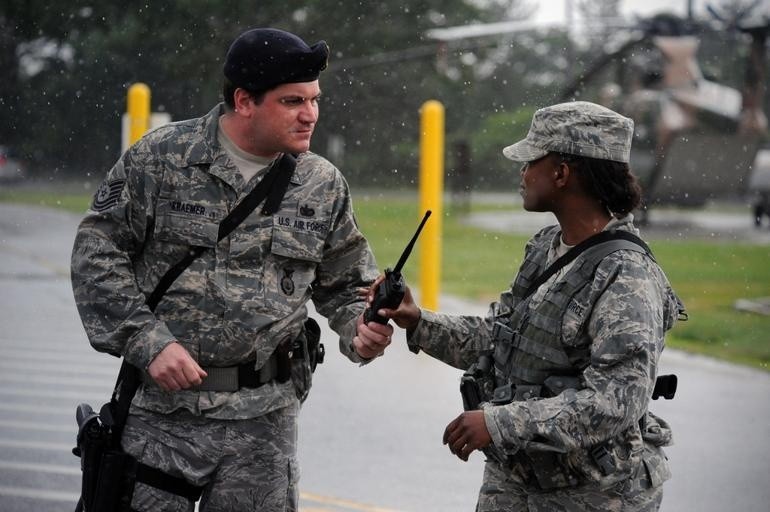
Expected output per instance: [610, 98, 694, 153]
[500, 99, 636, 168]
[221, 27, 331, 89]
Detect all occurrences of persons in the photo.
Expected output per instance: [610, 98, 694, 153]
[365, 101, 688, 512]
[63, 27, 395, 512]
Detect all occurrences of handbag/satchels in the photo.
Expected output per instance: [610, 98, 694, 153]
[73, 400, 113, 472]
[459, 354, 495, 414]
